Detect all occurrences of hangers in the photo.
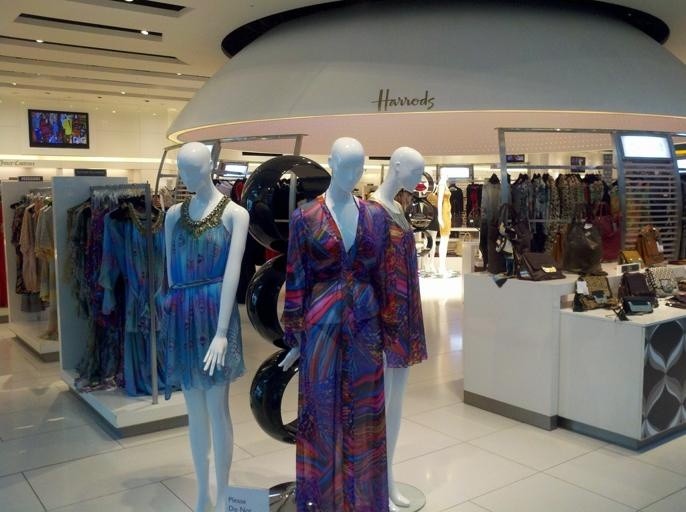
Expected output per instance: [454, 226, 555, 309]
[488, 165, 609, 185]
[448, 184, 462, 192]
[11, 183, 163, 219]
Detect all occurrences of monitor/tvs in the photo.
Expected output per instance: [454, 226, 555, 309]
[28, 109, 89, 148]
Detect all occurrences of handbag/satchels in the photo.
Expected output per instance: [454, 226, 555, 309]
[620, 251, 644, 271]
[520, 252, 565, 280]
[565, 220, 608, 275]
[622, 272, 659, 308]
[488, 219, 532, 275]
[636, 226, 664, 264]
[623, 302, 654, 314]
[648, 267, 679, 296]
[573, 275, 618, 312]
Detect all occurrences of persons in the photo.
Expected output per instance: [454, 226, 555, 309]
[436, 169, 456, 273]
[364, 146, 426, 512]
[280, 135, 402, 512]
[29, 111, 88, 146]
[154, 141, 250, 511]
